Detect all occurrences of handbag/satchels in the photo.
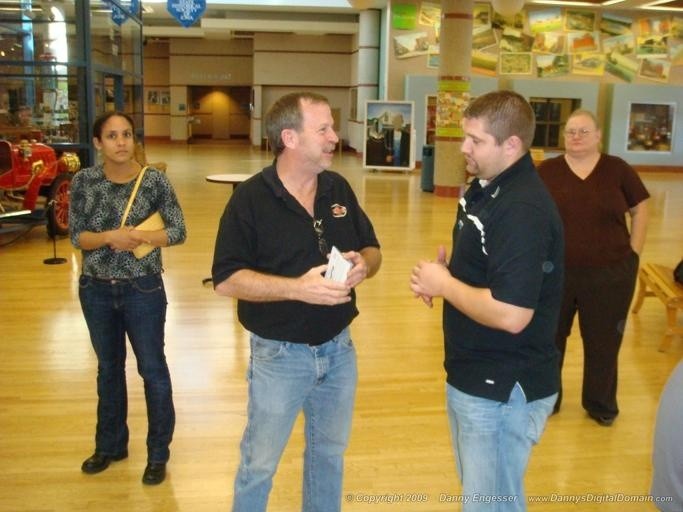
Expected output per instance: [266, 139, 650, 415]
[126, 211, 166, 259]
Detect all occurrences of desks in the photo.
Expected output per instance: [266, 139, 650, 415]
[202, 172, 257, 287]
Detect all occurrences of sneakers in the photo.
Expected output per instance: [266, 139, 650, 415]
[82, 450, 128, 473]
[143, 448, 170, 484]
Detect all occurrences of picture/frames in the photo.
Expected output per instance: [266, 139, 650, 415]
[362, 100, 416, 173]
[623, 100, 677, 156]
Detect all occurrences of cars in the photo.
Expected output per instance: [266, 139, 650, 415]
[0, 138, 77, 241]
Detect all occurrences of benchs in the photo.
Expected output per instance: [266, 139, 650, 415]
[632, 262, 682, 352]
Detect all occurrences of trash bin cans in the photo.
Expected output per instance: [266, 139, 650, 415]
[422, 144, 434, 192]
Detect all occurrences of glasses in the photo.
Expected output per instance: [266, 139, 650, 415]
[313, 219, 329, 258]
[565, 129, 592, 138]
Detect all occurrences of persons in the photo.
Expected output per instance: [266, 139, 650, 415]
[64, 111, 188, 485]
[211, 92, 384, 512]
[405, 89, 567, 511]
[534, 105, 652, 428]
